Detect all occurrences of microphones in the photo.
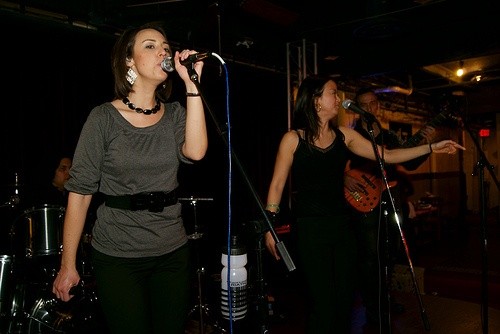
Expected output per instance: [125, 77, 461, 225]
[341, 99, 377, 122]
[161, 51, 215, 72]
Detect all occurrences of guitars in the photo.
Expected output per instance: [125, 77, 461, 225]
[343, 95, 456, 212]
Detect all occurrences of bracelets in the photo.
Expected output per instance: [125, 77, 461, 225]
[186, 93, 201, 96]
[263, 210, 276, 230]
[429, 144, 433, 152]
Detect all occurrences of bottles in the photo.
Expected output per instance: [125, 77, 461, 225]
[221, 236, 247, 320]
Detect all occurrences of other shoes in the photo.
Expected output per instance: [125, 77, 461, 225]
[365, 307, 392, 327]
[383, 294, 405, 315]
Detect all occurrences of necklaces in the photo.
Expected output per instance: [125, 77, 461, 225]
[122, 96, 161, 115]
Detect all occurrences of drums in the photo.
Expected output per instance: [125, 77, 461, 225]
[75, 233, 93, 276]
[23, 284, 106, 334]
[20, 205, 68, 257]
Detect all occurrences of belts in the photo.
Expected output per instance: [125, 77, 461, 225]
[105, 189, 178, 212]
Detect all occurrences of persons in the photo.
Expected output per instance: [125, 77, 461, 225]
[263, 75, 465, 334]
[344, 89, 435, 314]
[31, 155, 70, 206]
[52, 22, 208, 334]
